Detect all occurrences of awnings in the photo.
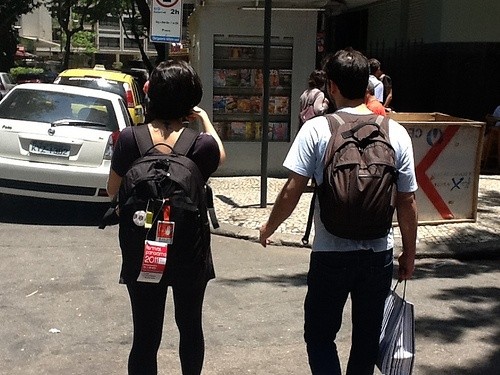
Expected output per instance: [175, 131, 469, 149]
[20, 35, 62, 60]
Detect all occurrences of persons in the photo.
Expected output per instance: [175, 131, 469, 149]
[259, 51, 419, 375]
[299, 70, 329, 123]
[367, 58, 392, 112]
[107, 59, 227, 375]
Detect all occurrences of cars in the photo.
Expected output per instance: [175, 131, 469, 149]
[0, 83, 135, 221]
[45, 63, 150, 126]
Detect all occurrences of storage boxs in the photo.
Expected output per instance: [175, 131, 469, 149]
[385, 112, 486, 227]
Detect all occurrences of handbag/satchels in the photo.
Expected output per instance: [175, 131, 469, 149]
[299, 91, 322, 124]
[373, 275, 416, 375]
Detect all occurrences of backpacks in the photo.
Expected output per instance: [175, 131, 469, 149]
[317, 112, 399, 240]
[117, 124, 217, 285]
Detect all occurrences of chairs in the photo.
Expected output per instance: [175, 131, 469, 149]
[77, 108, 107, 124]
